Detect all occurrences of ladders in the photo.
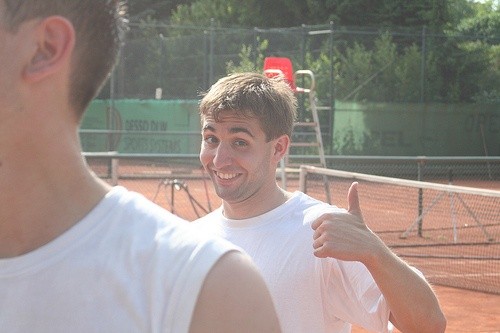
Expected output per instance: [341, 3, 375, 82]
[262, 67, 333, 207]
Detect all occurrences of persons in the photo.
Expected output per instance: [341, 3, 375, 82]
[188, 72, 448, 333]
[1, 0, 283, 333]
